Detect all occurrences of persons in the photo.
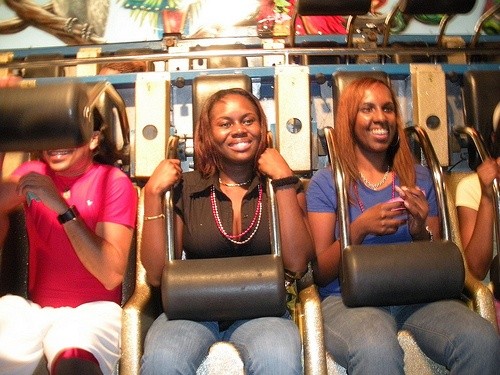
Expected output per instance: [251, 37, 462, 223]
[140, 88, 315, 375]
[455, 101, 500, 334]
[306, 77, 500, 375]
[0, 86, 139, 375]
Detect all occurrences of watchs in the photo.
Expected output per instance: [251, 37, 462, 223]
[57, 205, 80, 225]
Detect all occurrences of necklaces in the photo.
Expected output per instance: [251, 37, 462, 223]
[218, 175, 253, 187]
[356, 162, 390, 189]
[60, 176, 82, 189]
[353, 170, 395, 213]
[209, 183, 263, 245]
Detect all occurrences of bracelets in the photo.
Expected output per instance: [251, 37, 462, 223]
[144, 214, 166, 221]
[271, 176, 300, 188]
